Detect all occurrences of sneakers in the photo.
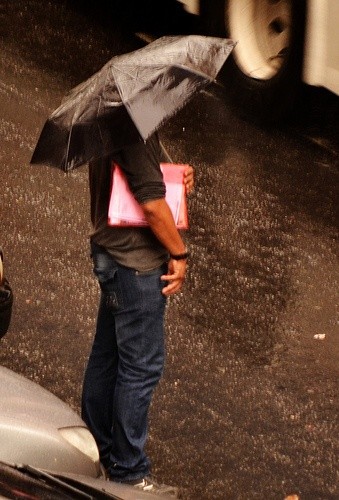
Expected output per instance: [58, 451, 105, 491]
[101, 463, 179, 500]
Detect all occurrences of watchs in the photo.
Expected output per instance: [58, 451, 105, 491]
[166, 253, 190, 263]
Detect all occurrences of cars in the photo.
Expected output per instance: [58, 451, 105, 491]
[0, 364, 110, 481]
[0, 460, 182, 500]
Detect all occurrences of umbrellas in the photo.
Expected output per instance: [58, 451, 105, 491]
[79, 130, 191, 496]
[30, 37, 239, 173]
